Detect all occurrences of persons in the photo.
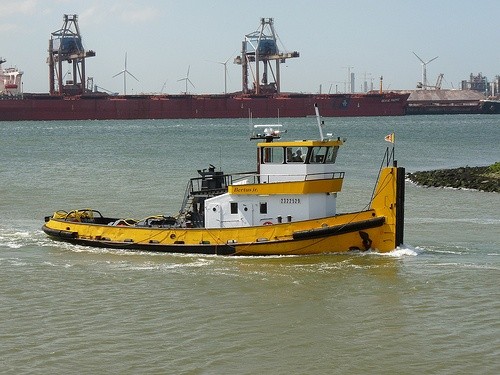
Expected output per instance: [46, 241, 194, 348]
[288, 150, 304, 162]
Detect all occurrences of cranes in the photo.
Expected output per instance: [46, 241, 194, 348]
[45, 14, 96, 99]
[232, 15, 300, 100]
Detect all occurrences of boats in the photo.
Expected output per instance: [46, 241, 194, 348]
[44, 102, 406, 256]
[0, 56, 24, 101]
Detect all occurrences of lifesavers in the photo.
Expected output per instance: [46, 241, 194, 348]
[263, 222, 273, 226]
